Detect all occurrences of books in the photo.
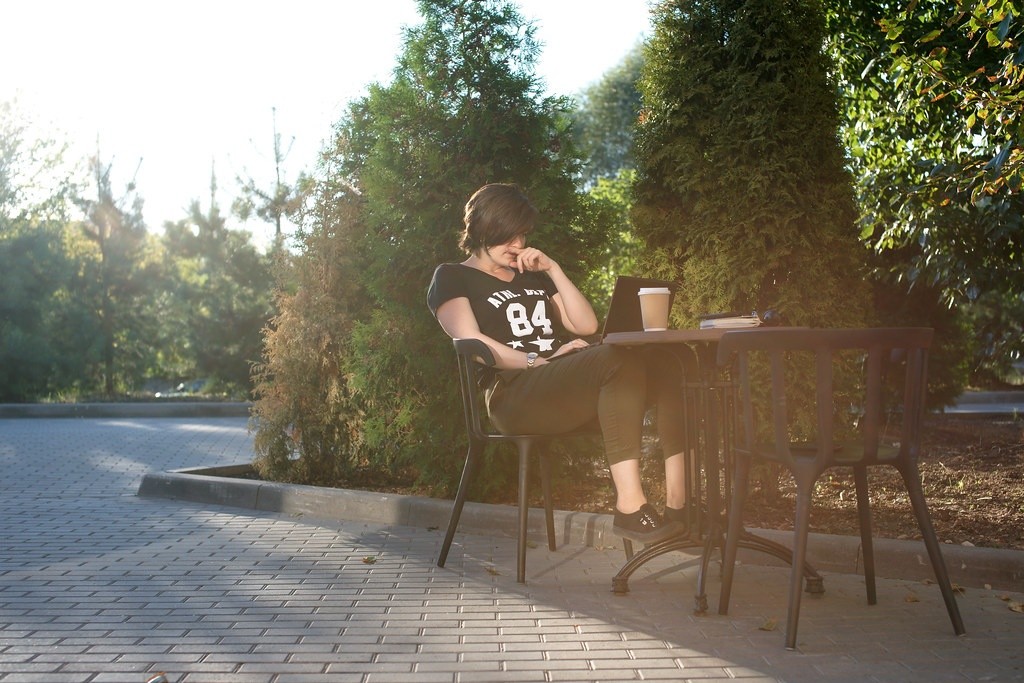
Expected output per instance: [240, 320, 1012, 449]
[699, 315, 760, 329]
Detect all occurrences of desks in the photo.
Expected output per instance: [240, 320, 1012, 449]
[601, 325, 809, 614]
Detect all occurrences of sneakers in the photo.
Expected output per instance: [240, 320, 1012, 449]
[612, 504, 684, 545]
[664, 501, 722, 532]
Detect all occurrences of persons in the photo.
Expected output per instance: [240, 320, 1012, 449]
[426, 184, 724, 546]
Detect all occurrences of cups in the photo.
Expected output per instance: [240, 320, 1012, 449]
[637, 287, 670, 331]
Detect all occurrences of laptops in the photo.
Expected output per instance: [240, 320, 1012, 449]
[547, 276, 679, 364]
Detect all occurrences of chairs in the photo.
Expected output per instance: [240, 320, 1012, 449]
[717, 326, 965, 649]
[439, 337, 599, 581]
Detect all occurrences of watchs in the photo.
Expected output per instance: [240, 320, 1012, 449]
[526, 351, 539, 370]
